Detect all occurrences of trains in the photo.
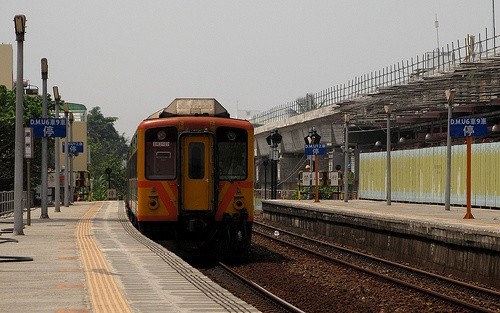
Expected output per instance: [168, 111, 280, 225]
[126, 98, 254, 256]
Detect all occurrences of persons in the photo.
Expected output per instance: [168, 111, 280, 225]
[343, 164, 354, 200]
[219, 151, 246, 178]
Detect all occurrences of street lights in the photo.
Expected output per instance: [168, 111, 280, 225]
[382, 102, 398, 205]
[63, 102, 69, 207]
[12, 12, 28, 234]
[39, 57, 49, 220]
[69, 112, 74, 203]
[53, 86, 61, 212]
[343, 112, 350, 202]
[444, 89, 455, 210]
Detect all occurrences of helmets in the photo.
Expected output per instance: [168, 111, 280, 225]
[305, 165, 310, 169]
[348, 164, 350, 169]
